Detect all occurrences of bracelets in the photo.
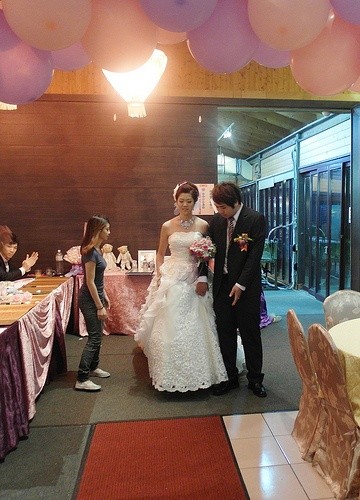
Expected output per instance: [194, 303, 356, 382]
[97, 306, 104, 310]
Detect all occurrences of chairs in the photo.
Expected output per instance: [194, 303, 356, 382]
[284, 290, 360, 500]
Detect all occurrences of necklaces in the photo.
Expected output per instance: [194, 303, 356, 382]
[176, 213, 195, 230]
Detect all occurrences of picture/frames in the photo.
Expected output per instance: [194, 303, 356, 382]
[137, 250, 157, 272]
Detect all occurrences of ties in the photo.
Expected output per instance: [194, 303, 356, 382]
[228, 216, 235, 242]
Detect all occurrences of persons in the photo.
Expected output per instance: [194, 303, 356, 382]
[0, 225, 38, 282]
[75, 216, 112, 391]
[196, 182, 266, 398]
[134, 183, 229, 393]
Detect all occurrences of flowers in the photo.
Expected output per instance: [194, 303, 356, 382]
[189, 236, 216, 267]
[234, 233, 253, 252]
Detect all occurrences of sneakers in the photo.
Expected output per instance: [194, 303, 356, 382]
[75, 380, 101, 390]
[89, 368, 110, 377]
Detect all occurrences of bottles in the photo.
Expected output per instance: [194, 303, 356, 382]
[55, 249, 64, 274]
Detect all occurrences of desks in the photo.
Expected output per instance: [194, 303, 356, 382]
[74, 269, 154, 335]
[0, 268, 77, 460]
[328, 317, 359, 428]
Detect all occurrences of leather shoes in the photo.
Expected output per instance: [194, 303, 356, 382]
[212, 381, 239, 396]
[248, 379, 267, 397]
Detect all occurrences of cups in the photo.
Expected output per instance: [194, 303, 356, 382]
[46, 268, 52, 277]
[34, 269, 42, 276]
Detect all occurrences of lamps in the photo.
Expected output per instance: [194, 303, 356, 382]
[101, 49, 167, 119]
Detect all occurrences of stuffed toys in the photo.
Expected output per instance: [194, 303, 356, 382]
[116, 246, 134, 270]
[103, 244, 116, 269]
